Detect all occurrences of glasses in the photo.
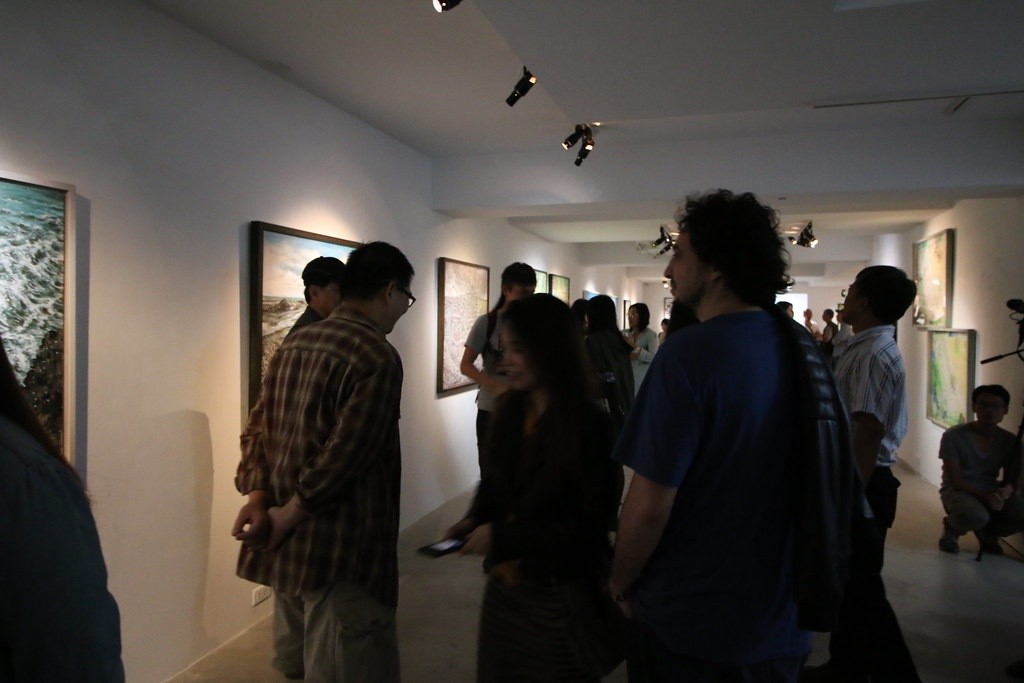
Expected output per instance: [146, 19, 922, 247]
[396, 286, 416, 307]
[842, 290, 855, 298]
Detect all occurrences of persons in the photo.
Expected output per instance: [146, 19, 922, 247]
[0, 337, 127, 683]
[658, 319, 669, 344]
[776, 301, 854, 368]
[801, 265, 924, 683]
[939, 384, 1024, 554]
[621, 302, 658, 396]
[230, 242, 414, 683]
[437, 294, 626, 683]
[460, 262, 536, 478]
[608, 192, 836, 683]
[573, 296, 633, 414]
[289, 256, 345, 335]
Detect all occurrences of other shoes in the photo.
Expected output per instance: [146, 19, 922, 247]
[973, 531, 1004, 554]
[939, 528, 960, 553]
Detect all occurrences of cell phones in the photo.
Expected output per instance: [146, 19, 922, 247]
[416, 538, 466, 558]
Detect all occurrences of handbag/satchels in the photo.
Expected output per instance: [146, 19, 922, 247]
[791, 318, 884, 633]
